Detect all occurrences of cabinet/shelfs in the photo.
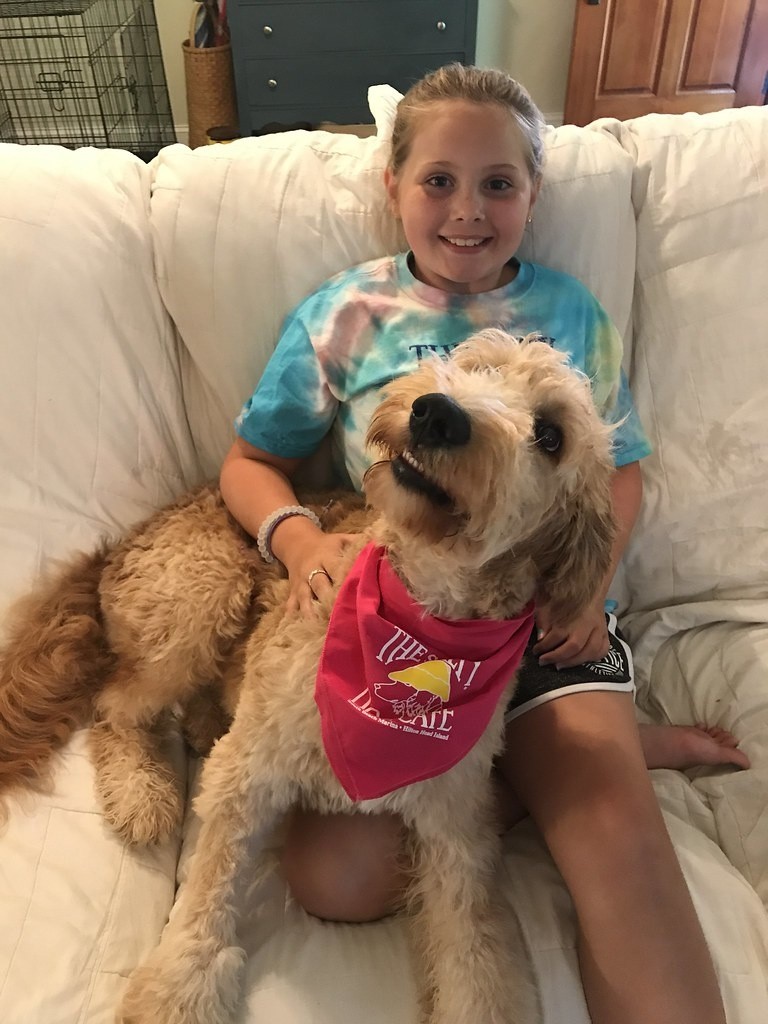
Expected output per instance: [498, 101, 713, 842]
[225, 0, 478, 137]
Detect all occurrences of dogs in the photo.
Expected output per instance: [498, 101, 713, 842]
[1, 326, 635, 1023]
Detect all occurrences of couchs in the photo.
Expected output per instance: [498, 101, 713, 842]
[0, 79, 768, 1023]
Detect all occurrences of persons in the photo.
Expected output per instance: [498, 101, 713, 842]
[210, 58, 750, 1024]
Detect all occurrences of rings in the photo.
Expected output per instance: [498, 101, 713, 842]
[308, 569, 327, 585]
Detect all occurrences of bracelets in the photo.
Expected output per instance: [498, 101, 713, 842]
[256, 504, 323, 565]
